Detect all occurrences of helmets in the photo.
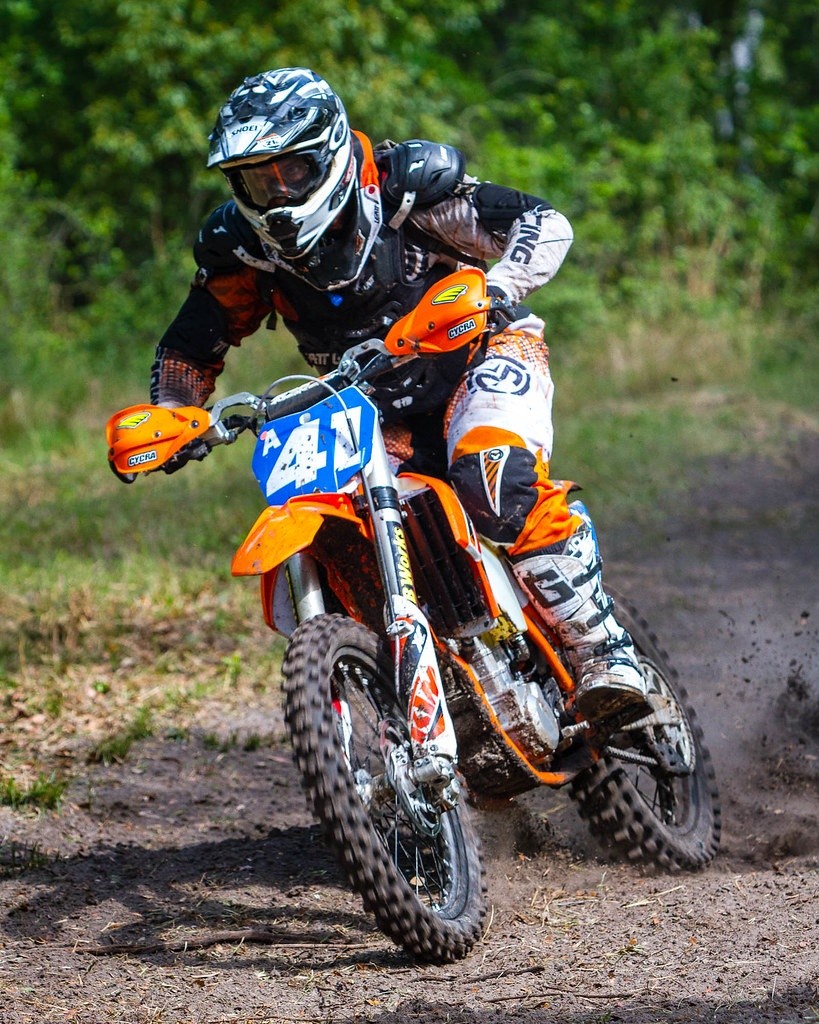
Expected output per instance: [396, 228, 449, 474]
[207, 67, 357, 259]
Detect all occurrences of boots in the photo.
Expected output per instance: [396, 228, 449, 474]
[512, 500, 647, 719]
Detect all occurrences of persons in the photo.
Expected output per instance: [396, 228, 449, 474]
[149, 69, 648, 727]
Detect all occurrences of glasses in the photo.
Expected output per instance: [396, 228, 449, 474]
[227, 150, 330, 211]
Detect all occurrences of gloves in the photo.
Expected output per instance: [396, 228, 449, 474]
[161, 439, 211, 474]
[487, 285, 515, 335]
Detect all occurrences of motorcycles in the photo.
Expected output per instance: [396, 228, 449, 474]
[109, 267, 719, 963]
